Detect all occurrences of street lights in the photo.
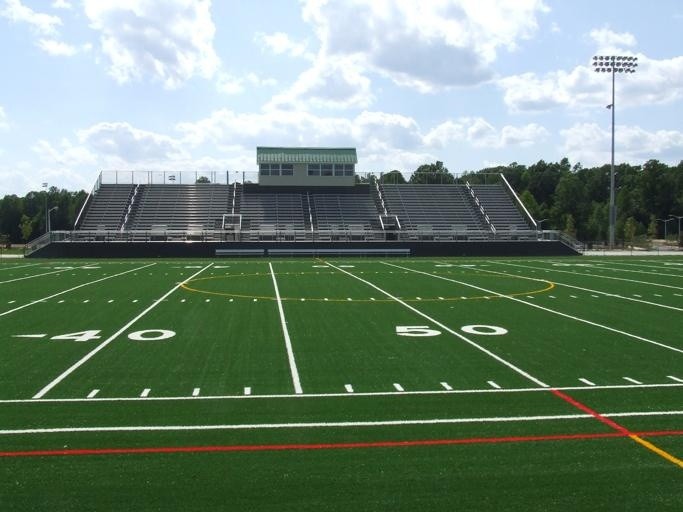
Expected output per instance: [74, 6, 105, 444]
[590, 51, 639, 250]
[47, 206, 58, 232]
